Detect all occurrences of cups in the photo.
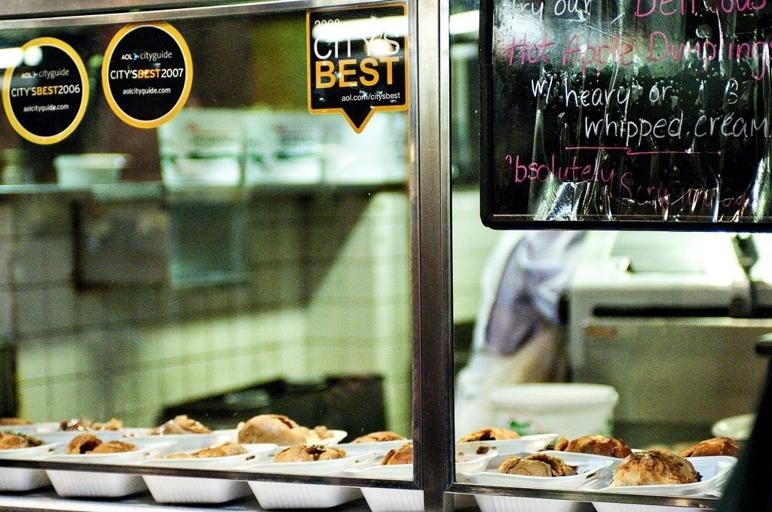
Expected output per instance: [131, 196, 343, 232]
[157, 105, 408, 188]
[485, 383, 620, 436]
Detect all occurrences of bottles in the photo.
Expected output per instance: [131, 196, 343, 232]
[1, 148, 36, 184]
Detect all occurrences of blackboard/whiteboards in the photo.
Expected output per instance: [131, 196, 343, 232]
[477, 0, 772, 232]
